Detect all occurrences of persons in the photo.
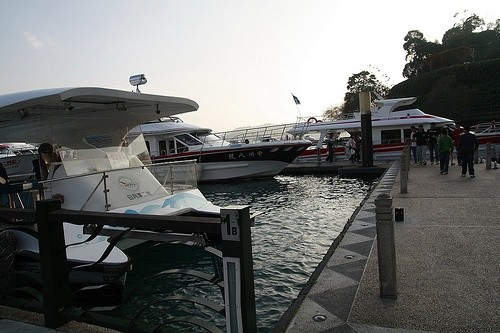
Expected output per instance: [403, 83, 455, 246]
[346, 133, 357, 162]
[438, 127, 453, 174]
[28, 142, 62, 181]
[411, 126, 418, 163]
[352, 132, 361, 162]
[326, 132, 334, 162]
[453, 123, 465, 163]
[458, 125, 479, 178]
[413, 124, 427, 165]
[426, 122, 440, 164]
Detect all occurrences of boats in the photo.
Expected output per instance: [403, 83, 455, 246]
[0, 73, 500, 311]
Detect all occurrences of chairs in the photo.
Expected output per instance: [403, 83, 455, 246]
[0, 163, 32, 209]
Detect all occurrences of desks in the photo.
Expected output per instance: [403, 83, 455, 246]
[8, 173, 36, 209]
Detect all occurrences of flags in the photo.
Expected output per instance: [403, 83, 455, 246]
[293, 94, 300, 104]
